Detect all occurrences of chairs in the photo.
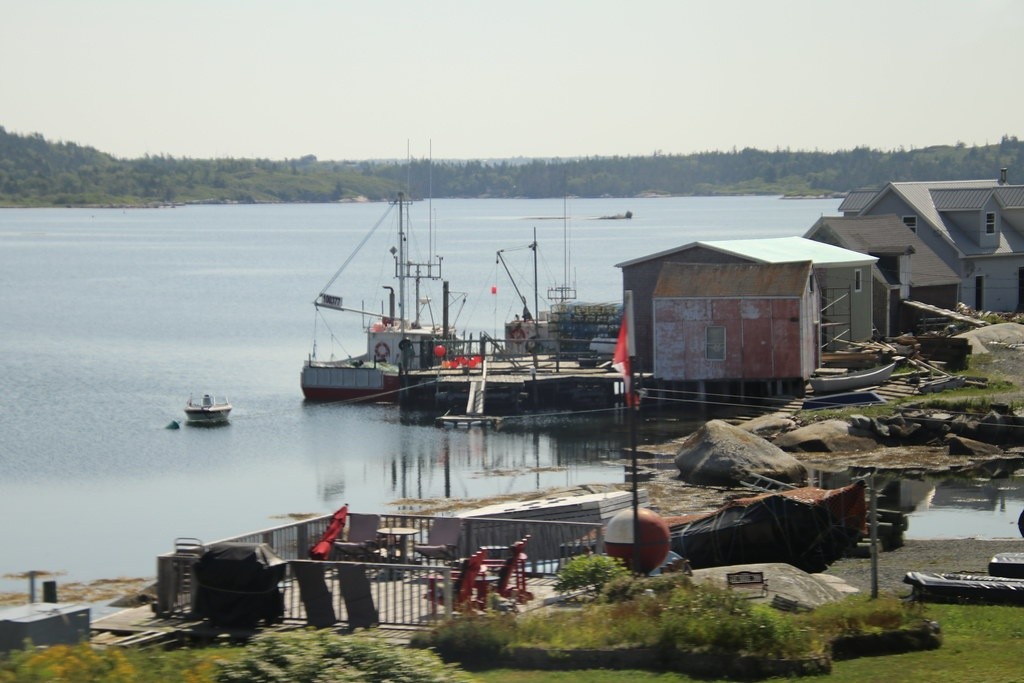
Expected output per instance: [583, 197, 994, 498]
[424, 535, 531, 615]
[414, 543, 461, 575]
[332, 538, 378, 579]
[481, 545, 511, 572]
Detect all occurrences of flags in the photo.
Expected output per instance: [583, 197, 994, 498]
[313, 506, 347, 559]
[613, 291, 639, 408]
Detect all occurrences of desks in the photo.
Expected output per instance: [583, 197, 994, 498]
[476, 576, 501, 607]
[377, 527, 420, 578]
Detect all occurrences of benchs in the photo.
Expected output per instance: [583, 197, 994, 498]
[727, 571, 768, 600]
[816, 368, 848, 376]
[483, 559, 508, 564]
[483, 564, 507, 571]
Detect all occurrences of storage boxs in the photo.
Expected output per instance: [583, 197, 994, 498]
[547, 302, 625, 367]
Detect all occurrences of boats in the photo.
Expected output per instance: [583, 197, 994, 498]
[802, 390, 888, 411]
[184, 393, 235, 424]
[808, 361, 898, 393]
[296, 139, 472, 399]
[557, 475, 874, 570]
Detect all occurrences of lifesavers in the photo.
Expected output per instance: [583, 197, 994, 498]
[510, 329, 526, 344]
[375, 342, 390, 358]
